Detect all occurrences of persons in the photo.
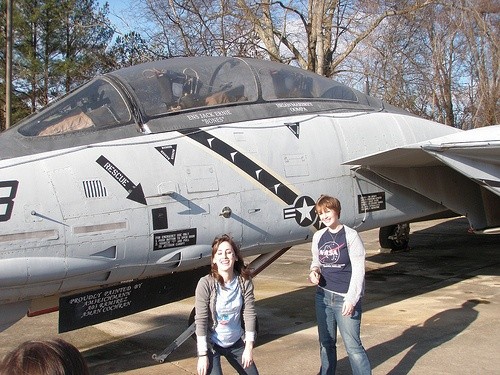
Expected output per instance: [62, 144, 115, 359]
[309, 195, 372, 375]
[193, 234, 260, 375]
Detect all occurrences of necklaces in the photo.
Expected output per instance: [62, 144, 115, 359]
[327, 224, 340, 244]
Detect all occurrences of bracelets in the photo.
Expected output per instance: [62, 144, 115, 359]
[198, 354, 207, 357]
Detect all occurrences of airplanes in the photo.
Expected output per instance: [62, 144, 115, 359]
[0, 56, 499, 340]
